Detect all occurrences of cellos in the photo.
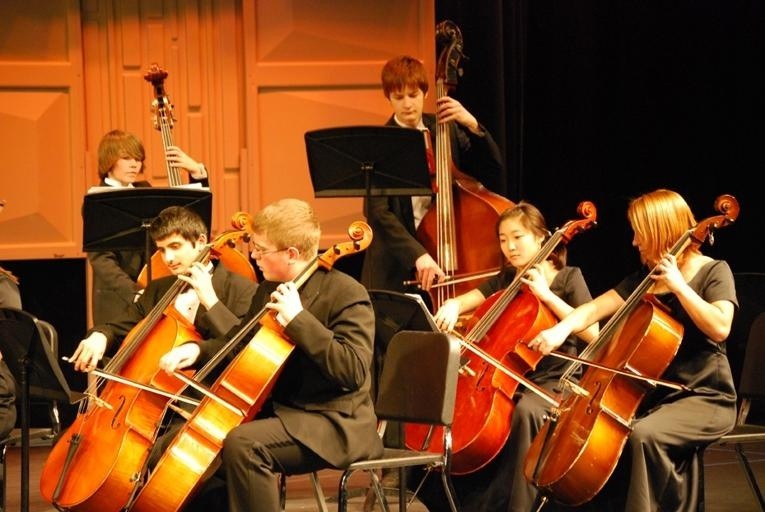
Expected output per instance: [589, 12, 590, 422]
[413, 21, 519, 315]
[402, 202, 597, 477]
[125, 217, 373, 512]
[136, 62, 257, 284]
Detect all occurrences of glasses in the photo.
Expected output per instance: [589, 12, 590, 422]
[247, 239, 290, 260]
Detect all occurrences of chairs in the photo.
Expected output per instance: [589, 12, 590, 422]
[1, 318, 61, 512]
[717, 315, 765, 512]
[279, 330, 461, 511]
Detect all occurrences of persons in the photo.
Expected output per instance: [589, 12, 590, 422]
[0, 264, 22, 443]
[432, 200, 601, 512]
[359, 57, 502, 488]
[87, 129, 209, 342]
[160, 198, 384, 512]
